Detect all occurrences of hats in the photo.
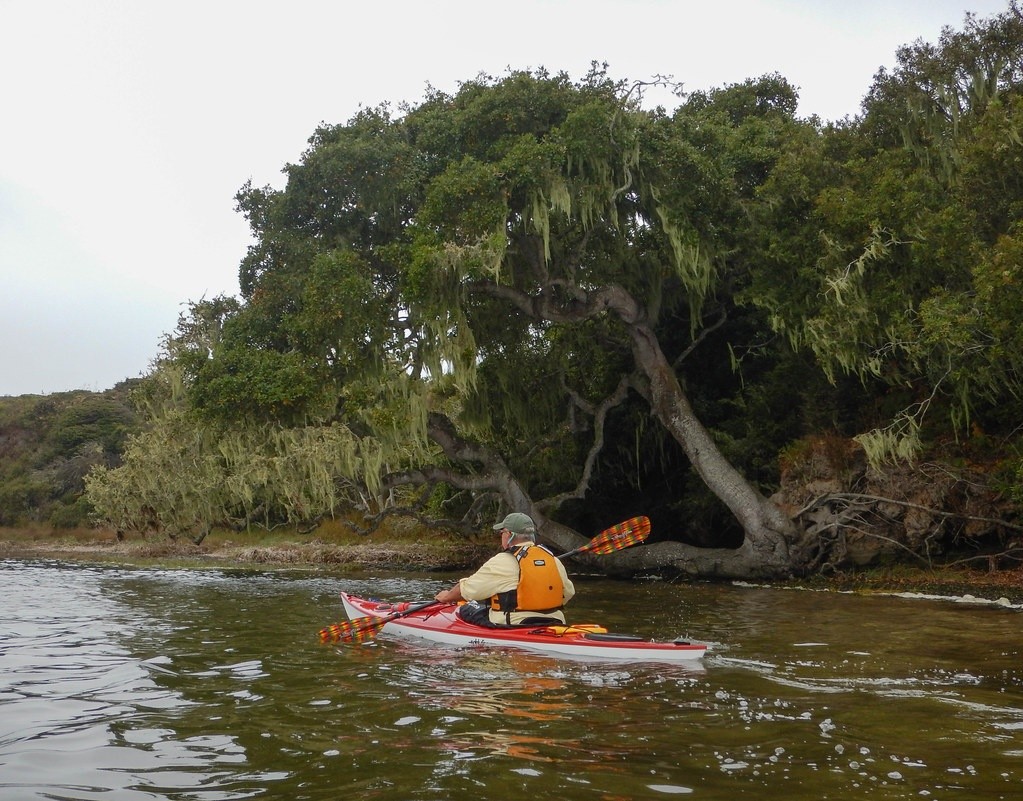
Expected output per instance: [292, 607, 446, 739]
[493, 513, 535, 534]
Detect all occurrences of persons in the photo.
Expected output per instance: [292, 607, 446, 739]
[434, 513, 576, 627]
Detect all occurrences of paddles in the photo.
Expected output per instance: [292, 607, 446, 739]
[316, 515, 652, 645]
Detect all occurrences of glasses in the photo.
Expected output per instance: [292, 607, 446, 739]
[500, 530, 510, 537]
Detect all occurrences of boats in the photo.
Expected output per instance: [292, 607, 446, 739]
[341, 592, 708, 663]
